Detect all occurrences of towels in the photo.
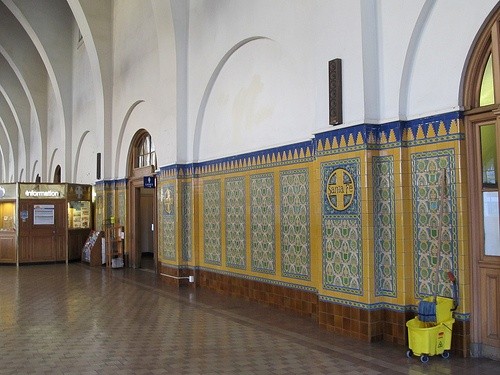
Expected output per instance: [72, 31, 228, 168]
[416, 299, 438, 324]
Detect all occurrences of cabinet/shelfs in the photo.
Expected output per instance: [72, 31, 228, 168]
[104, 225, 125, 271]
[81, 229, 105, 268]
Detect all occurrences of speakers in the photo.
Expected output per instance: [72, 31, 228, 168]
[329, 58, 343, 125]
